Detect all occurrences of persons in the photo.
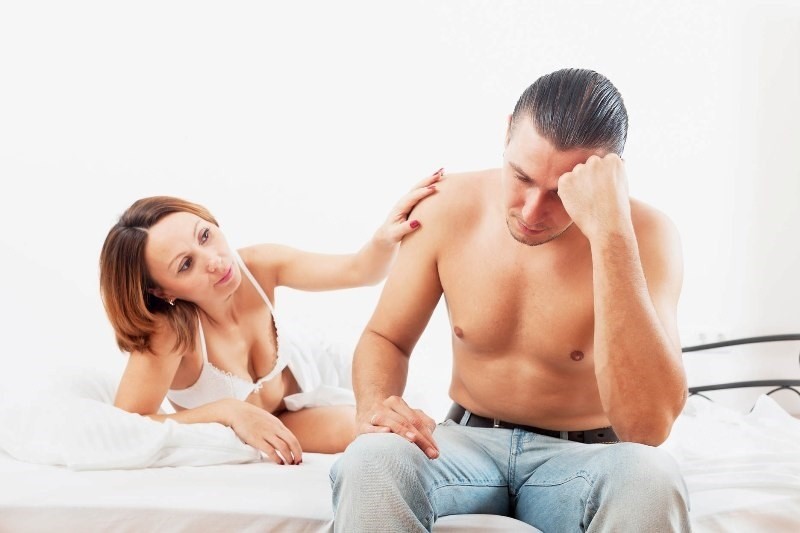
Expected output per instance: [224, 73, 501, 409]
[97, 166, 445, 465]
[327, 68, 694, 533]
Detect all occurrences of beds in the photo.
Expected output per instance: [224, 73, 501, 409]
[1, 330, 800, 533]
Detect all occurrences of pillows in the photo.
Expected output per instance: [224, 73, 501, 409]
[1, 385, 264, 469]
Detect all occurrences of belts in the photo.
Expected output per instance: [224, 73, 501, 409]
[447, 403, 620, 444]
